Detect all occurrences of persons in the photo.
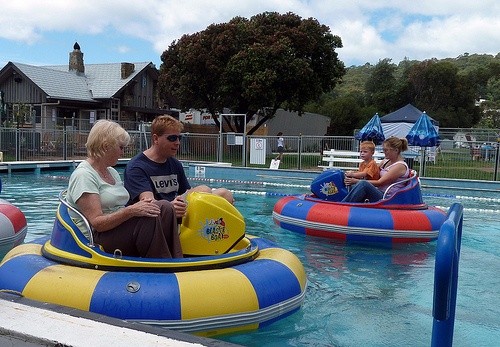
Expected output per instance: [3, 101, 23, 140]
[66, 119, 183, 260]
[317, 134, 330, 166]
[123, 115, 234, 229]
[338, 135, 409, 203]
[274, 132, 285, 163]
[345, 141, 380, 187]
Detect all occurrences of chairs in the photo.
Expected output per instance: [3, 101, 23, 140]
[50, 193, 106, 254]
[379, 168, 424, 204]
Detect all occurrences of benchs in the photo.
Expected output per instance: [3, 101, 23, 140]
[317, 149, 385, 171]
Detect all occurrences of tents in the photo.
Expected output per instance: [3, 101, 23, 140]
[380, 104, 438, 165]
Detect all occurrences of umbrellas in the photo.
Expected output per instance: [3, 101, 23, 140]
[405, 110, 439, 177]
[355, 112, 386, 145]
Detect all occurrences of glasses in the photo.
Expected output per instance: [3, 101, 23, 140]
[165, 135, 183, 142]
